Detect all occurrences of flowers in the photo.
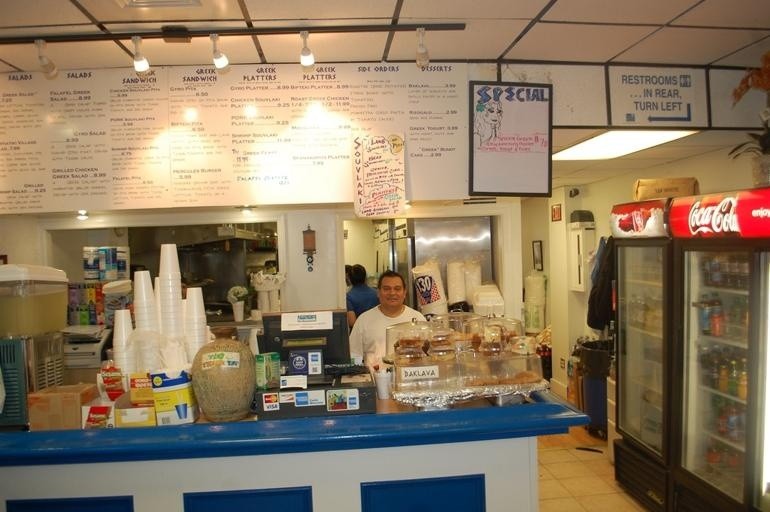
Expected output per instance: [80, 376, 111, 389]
[226, 285, 249, 305]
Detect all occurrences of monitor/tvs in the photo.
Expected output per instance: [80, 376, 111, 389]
[256, 307, 352, 388]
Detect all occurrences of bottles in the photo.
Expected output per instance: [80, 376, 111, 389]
[682, 254, 747, 490]
[625, 253, 661, 436]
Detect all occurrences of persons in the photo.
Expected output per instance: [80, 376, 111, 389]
[349, 270, 427, 367]
[473, 97, 503, 146]
[347, 264, 380, 335]
[345, 265, 353, 294]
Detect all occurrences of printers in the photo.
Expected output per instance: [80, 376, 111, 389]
[63, 329, 112, 368]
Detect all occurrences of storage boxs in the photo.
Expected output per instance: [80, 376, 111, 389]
[81, 371, 200, 429]
[632, 178, 697, 203]
[27, 383, 100, 430]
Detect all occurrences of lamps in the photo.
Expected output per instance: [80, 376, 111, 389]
[298, 30, 315, 67]
[131, 36, 150, 73]
[209, 33, 229, 71]
[34, 39, 58, 79]
[302, 224, 317, 273]
[415, 27, 430, 69]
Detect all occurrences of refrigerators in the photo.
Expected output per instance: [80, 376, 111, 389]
[672, 188, 770, 511]
[610, 200, 675, 512]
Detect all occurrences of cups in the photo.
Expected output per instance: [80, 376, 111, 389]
[374, 371, 394, 399]
[112, 243, 212, 379]
[250, 288, 282, 321]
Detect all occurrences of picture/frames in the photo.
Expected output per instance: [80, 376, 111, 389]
[532, 240, 543, 271]
[468, 80, 553, 198]
[551, 204, 561, 221]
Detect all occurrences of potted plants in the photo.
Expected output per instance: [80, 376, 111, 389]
[727, 118, 770, 189]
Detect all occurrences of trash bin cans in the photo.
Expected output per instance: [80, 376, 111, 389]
[582, 340, 614, 440]
